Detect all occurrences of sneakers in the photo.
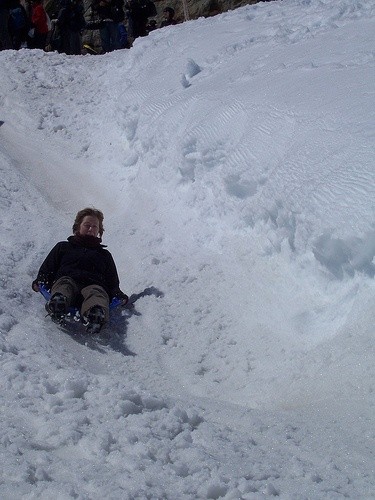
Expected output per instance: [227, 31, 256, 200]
[83, 304, 105, 333]
[45, 295, 69, 319]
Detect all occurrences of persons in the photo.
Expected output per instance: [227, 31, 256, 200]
[0, 0, 177, 55]
[32, 207, 129, 334]
